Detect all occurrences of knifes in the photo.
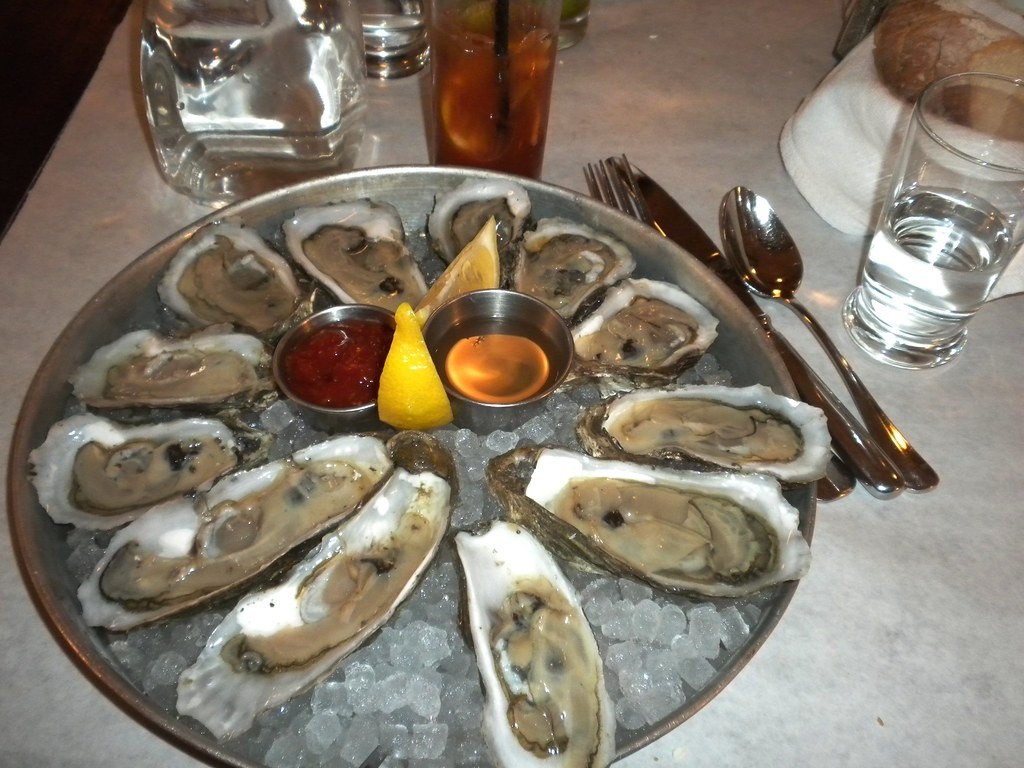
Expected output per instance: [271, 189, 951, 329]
[613, 157, 906, 498]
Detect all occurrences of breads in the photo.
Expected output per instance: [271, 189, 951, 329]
[872, 0, 1024, 145]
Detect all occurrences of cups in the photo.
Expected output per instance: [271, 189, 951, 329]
[426, 0, 562, 180]
[845, 72, 1024, 370]
[140, 0, 366, 210]
[357, 0, 430, 79]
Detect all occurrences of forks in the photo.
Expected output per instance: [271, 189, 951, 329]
[586, 157, 857, 499]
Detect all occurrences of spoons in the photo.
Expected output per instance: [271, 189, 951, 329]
[720, 188, 940, 495]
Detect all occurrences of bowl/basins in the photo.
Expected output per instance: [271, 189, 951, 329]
[418, 290, 574, 434]
[270, 304, 395, 433]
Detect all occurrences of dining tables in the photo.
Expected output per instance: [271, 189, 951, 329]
[1, 0, 1022, 767]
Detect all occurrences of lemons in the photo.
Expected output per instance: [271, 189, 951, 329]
[412, 214, 501, 329]
[375, 302, 455, 431]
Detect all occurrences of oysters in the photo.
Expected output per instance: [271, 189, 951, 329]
[31, 175, 830, 766]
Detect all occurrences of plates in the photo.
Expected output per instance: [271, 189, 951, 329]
[8, 166, 815, 768]
